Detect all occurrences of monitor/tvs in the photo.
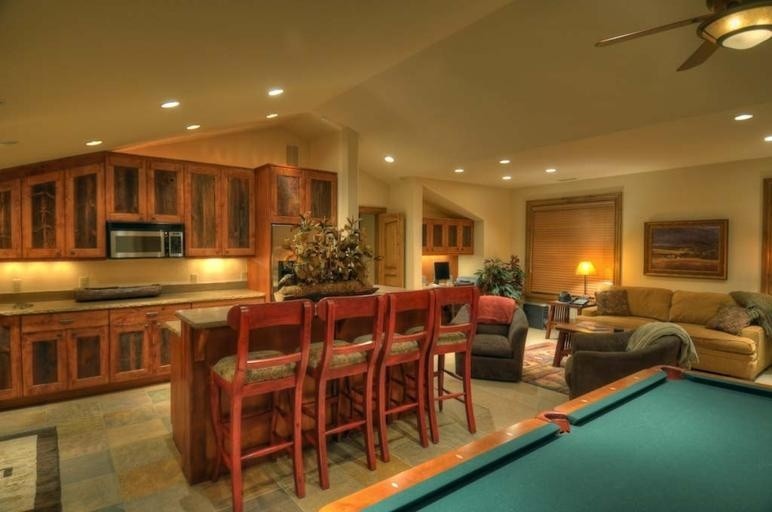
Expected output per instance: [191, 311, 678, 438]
[433, 261, 450, 285]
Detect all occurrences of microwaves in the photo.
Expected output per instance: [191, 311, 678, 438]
[109, 230, 184, 258]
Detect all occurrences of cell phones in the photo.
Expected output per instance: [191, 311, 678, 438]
[614, 328, 624, 332]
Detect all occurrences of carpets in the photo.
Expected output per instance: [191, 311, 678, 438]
[520, 342, 571, 395]
[0, 426, 63, 512]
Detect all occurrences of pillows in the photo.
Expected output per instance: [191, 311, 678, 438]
[594, 285, 754, 335]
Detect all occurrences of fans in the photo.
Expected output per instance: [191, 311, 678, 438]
[594, 0, 772, 72]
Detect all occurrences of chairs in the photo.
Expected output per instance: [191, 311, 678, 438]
[448, 296, 529, 382]
[564, 323, 683, 400]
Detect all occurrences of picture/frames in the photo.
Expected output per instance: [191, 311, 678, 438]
[643, 219, 729, 281]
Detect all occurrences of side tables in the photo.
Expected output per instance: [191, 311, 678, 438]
[545, 300, 597, 339]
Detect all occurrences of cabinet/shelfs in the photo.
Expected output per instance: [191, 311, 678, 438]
[255, 163, 337, 223]
[0, 150, 256, 263]
[0, 297, 265, 412]
[422, 217, 474, 256]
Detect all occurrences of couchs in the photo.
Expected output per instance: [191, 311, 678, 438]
[575, 286, 772, 381]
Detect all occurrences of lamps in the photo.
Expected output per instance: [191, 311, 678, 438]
[696, 2, 772, 50]
[575, 261, 597, 295]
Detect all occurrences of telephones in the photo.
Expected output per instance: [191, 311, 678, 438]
[570, 297, 588, 305]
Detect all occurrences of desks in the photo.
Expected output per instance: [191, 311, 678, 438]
[317, 365, 772, 512]
